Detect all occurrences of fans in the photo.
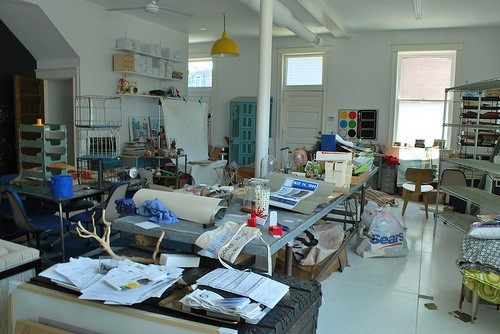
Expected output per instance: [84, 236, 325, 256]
[105, 0, 195, 17]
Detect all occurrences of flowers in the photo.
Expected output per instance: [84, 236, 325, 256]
[385, 154, 400, 167]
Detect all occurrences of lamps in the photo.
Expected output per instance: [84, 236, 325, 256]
[211, 14, 240, 58]
[414, 0, 421, 21]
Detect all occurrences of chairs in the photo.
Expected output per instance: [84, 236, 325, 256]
[402, 168, 433, 219]
[0, 173, 130, 276]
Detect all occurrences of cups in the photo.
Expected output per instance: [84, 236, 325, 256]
[243, 182, 257, 210]
[256, 186, 270, 215]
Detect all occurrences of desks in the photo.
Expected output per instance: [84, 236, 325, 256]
[219, 152, 228, 160]
[11, 180, 112, 263]
[0, 240, 322, 334]
[112, 166, 379, 283]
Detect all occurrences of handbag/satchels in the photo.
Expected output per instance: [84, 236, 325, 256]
[355, 202, 410, 259]
[291, 223, 344, 265]
[194, 220, 260, 263]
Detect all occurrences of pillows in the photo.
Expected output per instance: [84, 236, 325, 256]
[467, 222, 500, 239]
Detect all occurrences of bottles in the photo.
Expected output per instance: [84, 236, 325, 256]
[279, 147, 307, 174]
[261, 155, 277, 180]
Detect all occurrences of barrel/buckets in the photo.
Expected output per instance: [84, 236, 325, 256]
[51, 175, 74, 197]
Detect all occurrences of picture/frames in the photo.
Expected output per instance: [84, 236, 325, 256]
[128, 115, 163, 142]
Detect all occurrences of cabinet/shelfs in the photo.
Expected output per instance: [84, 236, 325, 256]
[115, 47, 183, 100]
[433, 77, 500, 235]
[381, 168, 396, 195]
[13, 74, 44, 170]
[18, 123, 67, 193]
[144, 155, 187, 189]
[229, 96, 272, 164]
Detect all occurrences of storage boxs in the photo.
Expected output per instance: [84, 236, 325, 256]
[316, 151, 369, 190]
[160, 254, 201, 268]
[404, 189, 444, 204]
[276, 246, 348, 283]
